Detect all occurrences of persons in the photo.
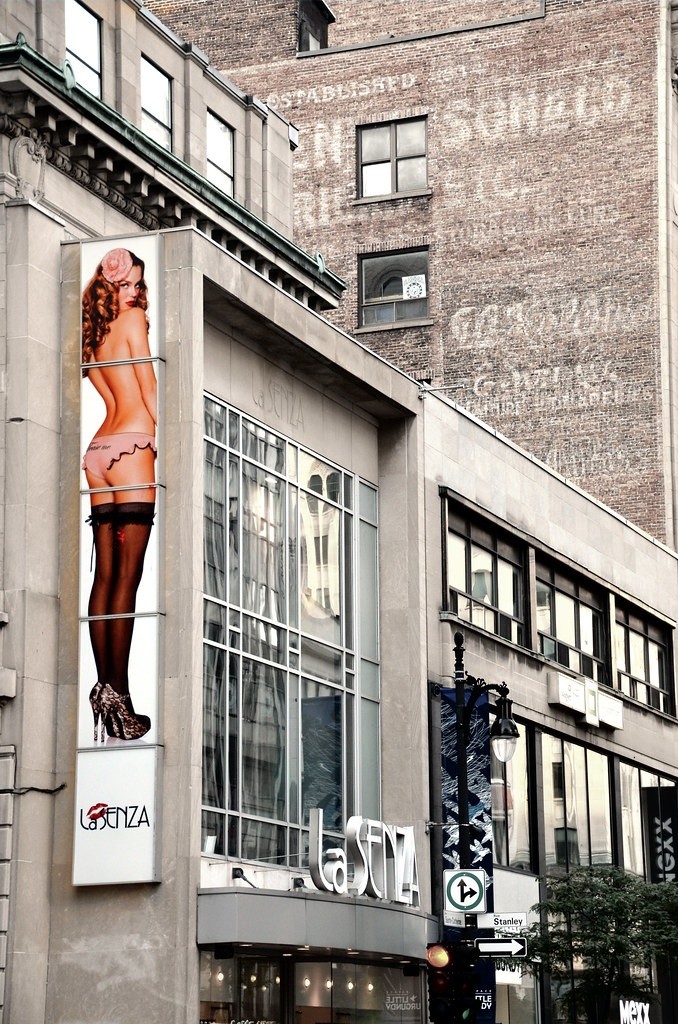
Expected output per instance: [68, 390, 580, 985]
[81, 248, 157, 743]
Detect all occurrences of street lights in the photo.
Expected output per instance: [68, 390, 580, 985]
[429, 629, 519, 926]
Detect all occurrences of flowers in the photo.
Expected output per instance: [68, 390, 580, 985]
[101, 249, 132, 284]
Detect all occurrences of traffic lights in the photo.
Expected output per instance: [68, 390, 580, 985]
[426, 943, 451, 968]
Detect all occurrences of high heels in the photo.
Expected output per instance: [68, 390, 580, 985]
[89, 683, 120, 742]
[98, 683, 151, 742]
